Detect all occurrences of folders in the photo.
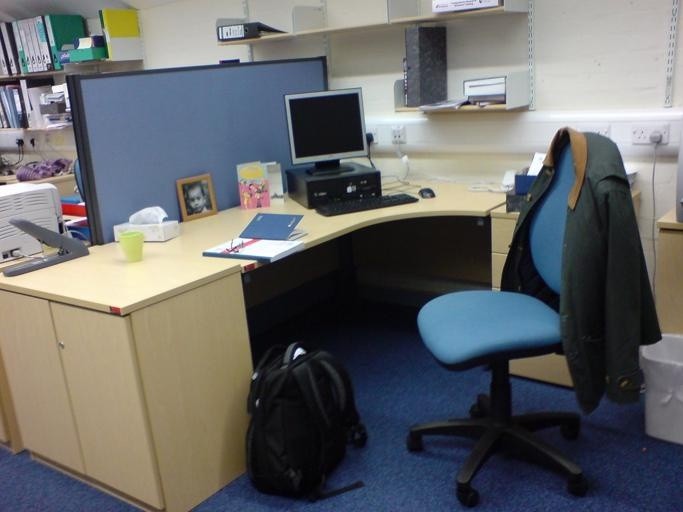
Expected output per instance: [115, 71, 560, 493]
[217, 22, 287, 41]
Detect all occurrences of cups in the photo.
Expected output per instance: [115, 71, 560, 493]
[119, 231, 143, 264]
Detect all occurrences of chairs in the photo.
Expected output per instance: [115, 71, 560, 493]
[407, 136, 587, 508]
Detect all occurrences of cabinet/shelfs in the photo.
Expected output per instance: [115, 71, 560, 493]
[654, 205, 683, 334]
[0, 0, 144, 130]
[0, 182, 641, 512]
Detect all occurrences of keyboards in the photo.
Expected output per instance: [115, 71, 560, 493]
[316, 194, 419, 217]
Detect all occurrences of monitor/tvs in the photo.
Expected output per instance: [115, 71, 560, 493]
[283, 87, 368, 177]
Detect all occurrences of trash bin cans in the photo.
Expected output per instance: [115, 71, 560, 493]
[638, 333, 683, 445]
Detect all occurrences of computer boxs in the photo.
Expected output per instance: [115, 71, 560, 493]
[287, 161, 381, 209]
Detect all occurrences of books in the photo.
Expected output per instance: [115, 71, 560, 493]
[419, 100, 465, 110]
[238, 213, 308, 242]
[203, 237, 305, 263]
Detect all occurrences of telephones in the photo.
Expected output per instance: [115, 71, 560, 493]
[501, 170, 516, 193]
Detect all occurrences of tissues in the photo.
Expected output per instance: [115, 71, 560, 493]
[112, 206, 180, 243]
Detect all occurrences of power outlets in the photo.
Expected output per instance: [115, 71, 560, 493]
[631, 125, 669, 144]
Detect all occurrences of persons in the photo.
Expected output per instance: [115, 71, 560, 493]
[187, 182, 208, 215]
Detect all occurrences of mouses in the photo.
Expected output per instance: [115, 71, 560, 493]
[420, 188, 435, 198]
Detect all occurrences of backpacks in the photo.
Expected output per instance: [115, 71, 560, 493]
[241, 339, 370, 504]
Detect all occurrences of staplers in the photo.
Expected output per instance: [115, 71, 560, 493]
[3, 218, 89, 277]
